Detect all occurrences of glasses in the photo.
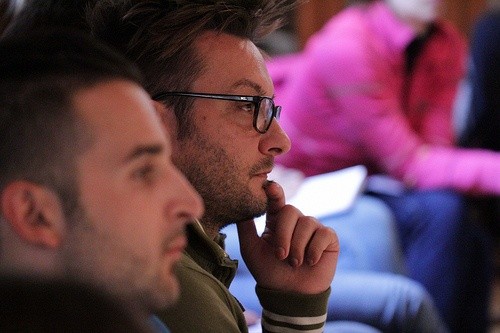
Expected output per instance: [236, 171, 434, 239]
[151, 91, 282, 134]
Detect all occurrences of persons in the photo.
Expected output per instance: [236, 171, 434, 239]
[0, 28, 205, 332]
[455, 8, 500, 237]
[0, 0, 339, 333]
[266, 0, 500, 329]
[228, 273, 448, 333]
